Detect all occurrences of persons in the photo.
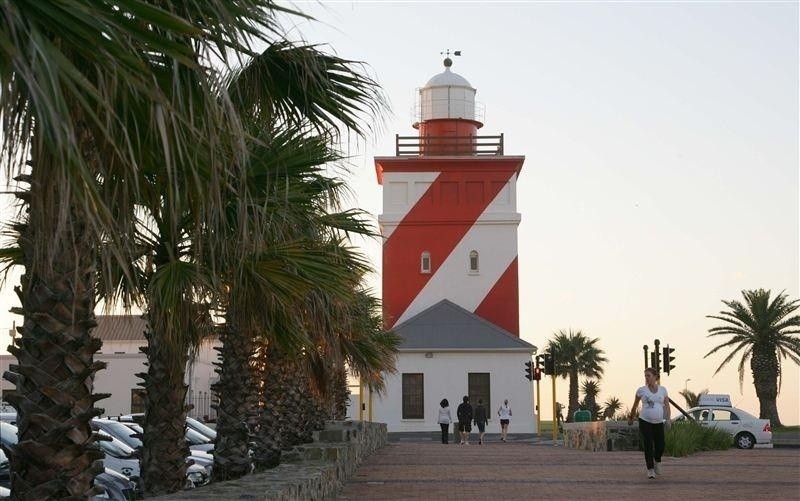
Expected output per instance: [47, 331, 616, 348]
[456, 396, 472, 445]
[702, 412, 708, 421]
[626, 366, 672, 480]
[437, 399, 453, 444]
[474, 397, 489, 444]
[497, 399, 513, 442]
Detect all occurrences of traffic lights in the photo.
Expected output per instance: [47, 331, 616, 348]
[539, 354, 553, 375]
[535, 368, 541, 380]
[524, 361, 532, 381]
[651, 352, 662, 374]
[663, 345, 676, 376]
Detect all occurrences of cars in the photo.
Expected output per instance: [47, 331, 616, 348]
[666, 394, 772, 450]
[0, 411, 264, 501]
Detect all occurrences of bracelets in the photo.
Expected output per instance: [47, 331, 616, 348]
[666, 419, 671, 422]
[629, 417, 634, 419]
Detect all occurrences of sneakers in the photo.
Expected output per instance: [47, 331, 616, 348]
[655, 463, 664, 475]
[442, 437, 508, 446]
[647, 469, 656, 479]
